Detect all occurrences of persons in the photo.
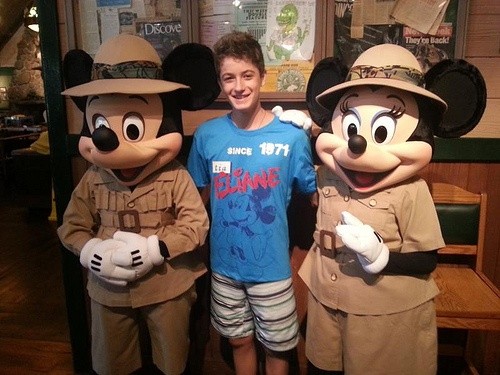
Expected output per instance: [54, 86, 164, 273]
[187, 32, 319, 375]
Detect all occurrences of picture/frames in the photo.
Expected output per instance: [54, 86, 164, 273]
[64, 0, 191, 63]
[191, 0, 327, 102]
[325, 0, 471, 86]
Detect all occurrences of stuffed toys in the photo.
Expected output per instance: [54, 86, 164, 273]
[275, 42, 487, 374]
[55, 33, 223, 375]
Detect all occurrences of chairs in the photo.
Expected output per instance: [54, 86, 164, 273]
[429, 181, 500, 375]
[16, 155, 51, 223]
